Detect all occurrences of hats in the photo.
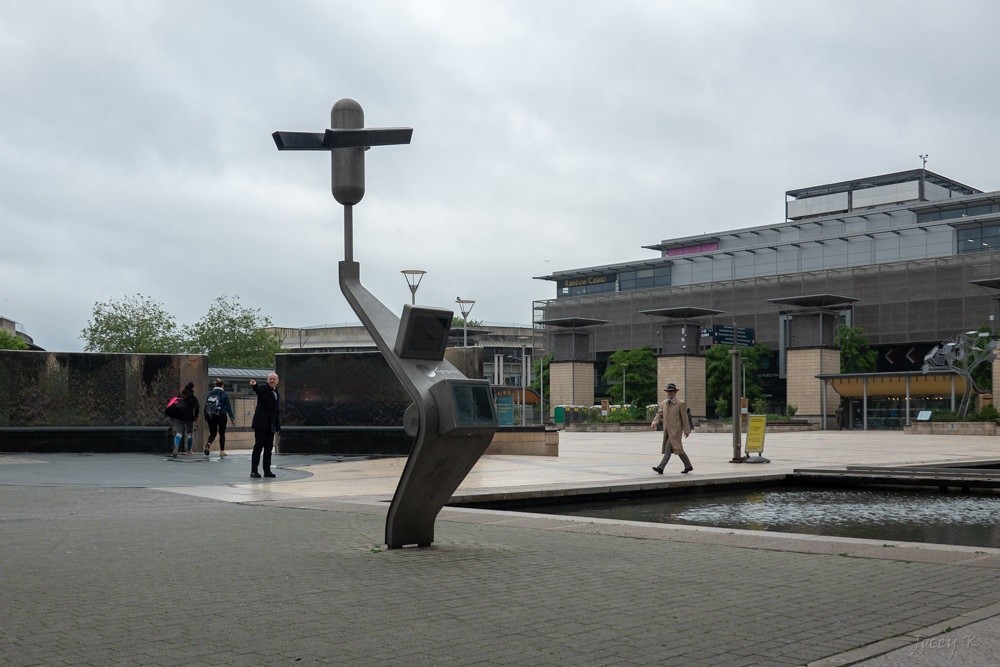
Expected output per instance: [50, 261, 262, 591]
[663, 383, 680, 391]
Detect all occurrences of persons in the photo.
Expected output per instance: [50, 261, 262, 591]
[686, 408, 694, 429]
[249, 373, 281, 478]
[651, 383, 694, 475]
[172, 381, 199, 457]
[204, 378, 235, 457]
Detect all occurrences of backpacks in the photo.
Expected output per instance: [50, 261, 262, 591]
[205, 390, 225, 419]
[165, 394, 189, 419]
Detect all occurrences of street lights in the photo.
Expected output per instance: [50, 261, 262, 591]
[455, 301, 476, 347]
[401, 270, 427, 304]
[740, 358, 749, 397]
[517, 336, 531, 426]
[621, 364, 629, 404]
[536, 349, 547, 424]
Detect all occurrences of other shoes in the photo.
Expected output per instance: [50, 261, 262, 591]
[173, 449, 178, 458]
[220, 451, 227, 457]
[204, 443, 210, 455]
[263, 472, 276, 477]
[681, 466, 693, 473]
[187, 450, 193, 456]
[250, 472, 261, 478]
[652, 466, 664, 474]
